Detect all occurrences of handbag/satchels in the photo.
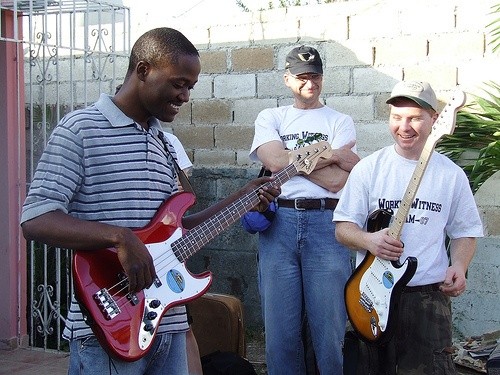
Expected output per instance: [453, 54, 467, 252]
[239, 200, 276, 234]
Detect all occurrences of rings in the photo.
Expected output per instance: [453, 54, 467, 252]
[457, 291, 460, 295]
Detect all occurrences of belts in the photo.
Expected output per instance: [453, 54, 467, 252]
[277, 198, 340, 211]
[403, 284, 443, 292]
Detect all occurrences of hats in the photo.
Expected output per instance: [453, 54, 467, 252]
[284, 45, 323, 75]
[386, 80, 437, 113]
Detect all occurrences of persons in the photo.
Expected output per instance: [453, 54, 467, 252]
[249, 46, 360, 375]
[20, 28, 281, 375]
[332, 79, 481, 375]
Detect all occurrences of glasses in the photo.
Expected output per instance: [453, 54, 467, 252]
[289, 73, 322, 82]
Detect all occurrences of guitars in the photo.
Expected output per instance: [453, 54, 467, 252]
[73, 142, 334, 360]
[342, 89, 468, 341]
